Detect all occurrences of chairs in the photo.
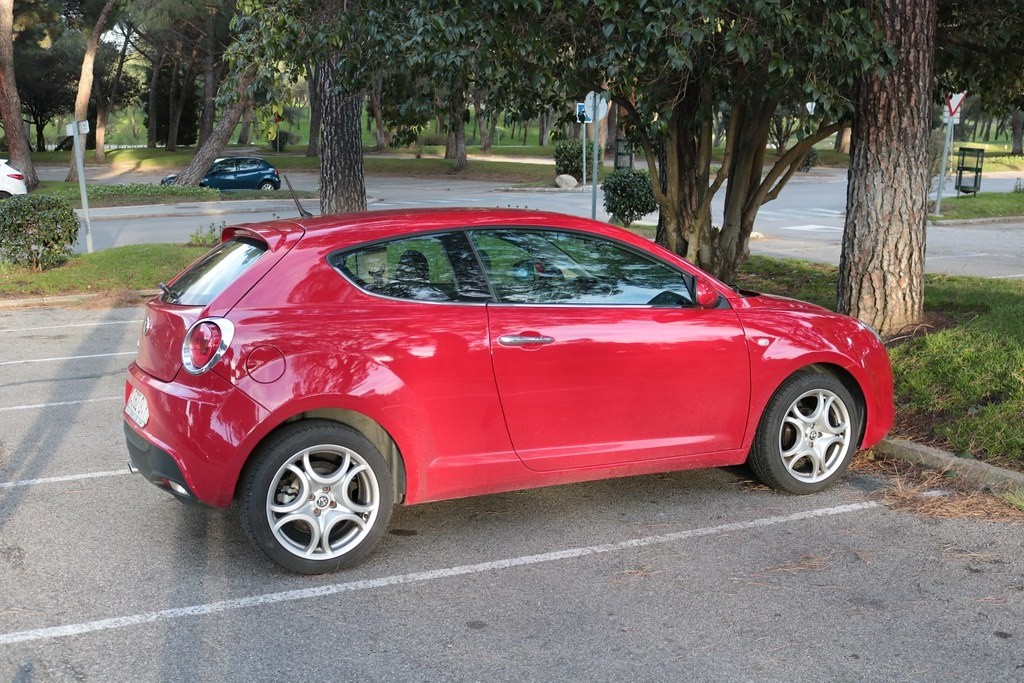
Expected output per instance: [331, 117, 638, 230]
[384, 249, 452, 303]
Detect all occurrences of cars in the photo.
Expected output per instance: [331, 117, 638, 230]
[0, 158, 27, 202]
[160, 155, 281, 192]
[122, 204, 895, 578]
